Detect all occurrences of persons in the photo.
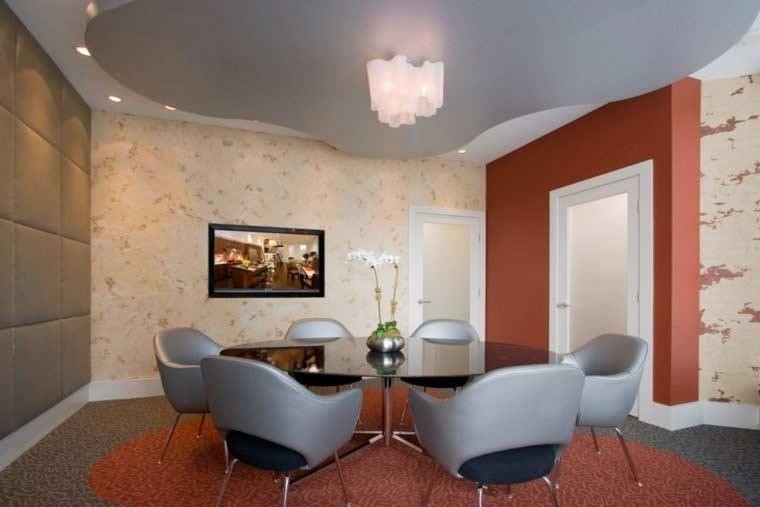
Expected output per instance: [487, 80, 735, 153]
[303, 251, 317, 265]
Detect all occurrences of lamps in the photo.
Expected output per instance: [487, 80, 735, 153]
[366, 56, 445, 128]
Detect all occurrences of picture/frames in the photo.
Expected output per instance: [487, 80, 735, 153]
[208, 222, 325, 297]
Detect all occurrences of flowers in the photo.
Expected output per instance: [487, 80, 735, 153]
[345, 249, 401, 334]
